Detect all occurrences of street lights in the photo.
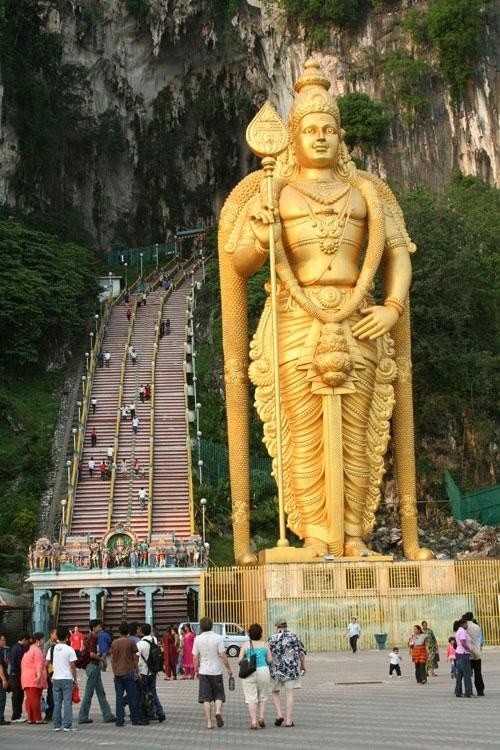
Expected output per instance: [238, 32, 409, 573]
[187, 270, 206, 484]
[174, 235, 178, 254]
[155, 243, 160, 269]
[199, 497, 209, 544]
[201, 256, 206, 278]
[59, 269, 117, 537]
[124, 261, 130, 293]
[139, 252, 144, 277]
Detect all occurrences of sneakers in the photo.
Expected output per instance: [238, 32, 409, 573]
[0, 714, 165, 732]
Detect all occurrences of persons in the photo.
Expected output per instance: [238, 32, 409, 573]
[191, 616, 234, 730]
[264, 617, 308, 728]
[386, 647, 403, 679]
[230, 56, 414, 563]
[343, 617, 362, 656]
[2, 609, 197, 735]
[421, 621, 439, 677]
[26, 534, 208, 573]
[407, 625, 430, 685]
[85, 239, 207, 512]
[444, 610, 486, 699]
[237, 622, 275, 730]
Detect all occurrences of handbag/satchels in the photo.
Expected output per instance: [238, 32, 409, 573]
[75, 653, 91, 669]
[41, 698, 50, 712]
[239, 655, 256, 677]
[48, 664, 53, 683]
[137, 680, 158, 719]
[6, 675, 18, 692]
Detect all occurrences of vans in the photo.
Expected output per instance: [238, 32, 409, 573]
[176, 620, 250, 658]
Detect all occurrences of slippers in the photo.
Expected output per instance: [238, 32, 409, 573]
[207, 713, 295, 729]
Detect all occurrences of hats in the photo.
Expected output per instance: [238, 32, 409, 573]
[275, 618, 287, 627]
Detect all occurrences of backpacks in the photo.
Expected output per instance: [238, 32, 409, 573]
[140, 637, 164, 672]
